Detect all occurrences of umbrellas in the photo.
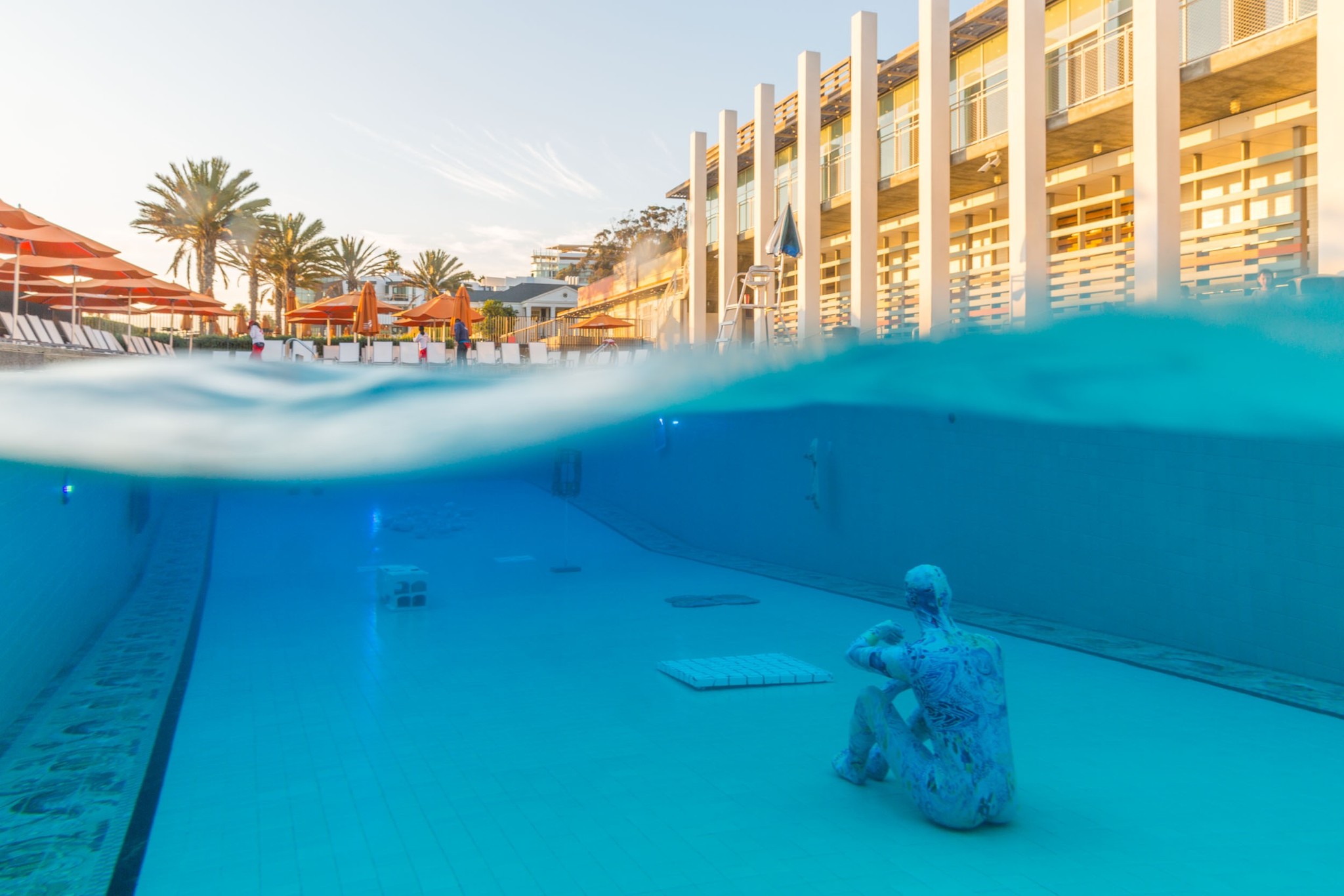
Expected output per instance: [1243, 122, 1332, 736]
[763, 202, 804, 309]
[412, 325, 432, 367]
[0, 196, 237, 355]
[566, 312, 638, 352]
[280, 278, 485, 361]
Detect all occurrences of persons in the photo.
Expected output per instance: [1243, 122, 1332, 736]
[1250, 268, 1281, 302]
[453, 317, 471, 374]
[829, 562, 1016, 830]
[247, 320, 266, 362]
[1180, 284, 1203, 307]
